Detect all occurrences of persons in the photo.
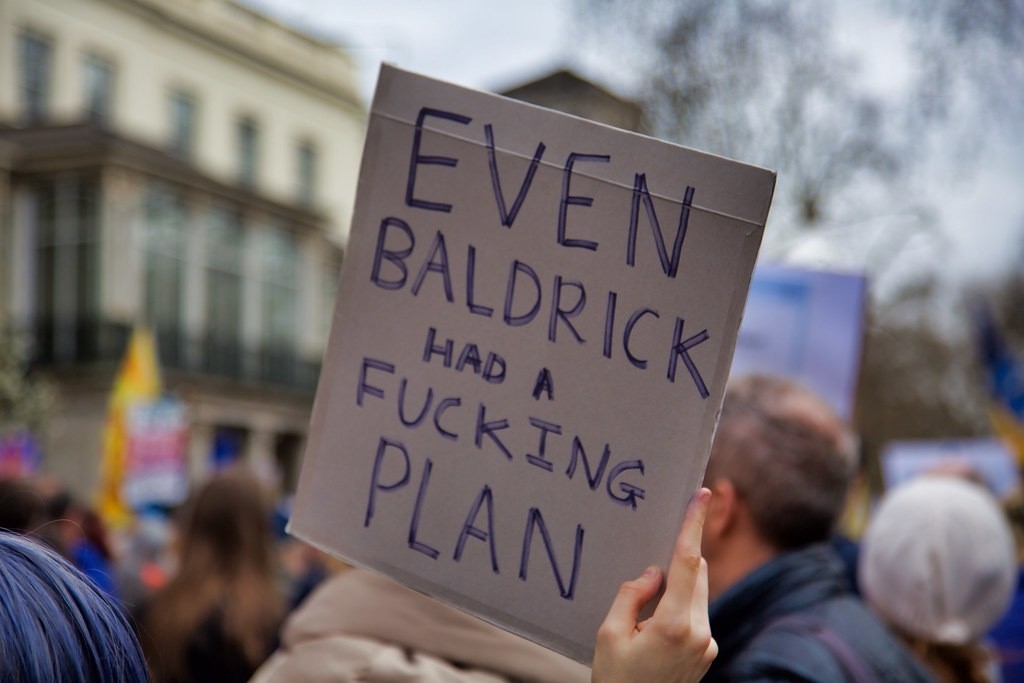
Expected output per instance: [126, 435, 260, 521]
[0, 488, 718, 683]
[835, 462, 1024, 683]
[1, 468, 591, 682]
[701, 373, 938, 683]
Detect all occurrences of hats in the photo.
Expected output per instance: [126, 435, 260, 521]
[858, 475, 1015, 643]
